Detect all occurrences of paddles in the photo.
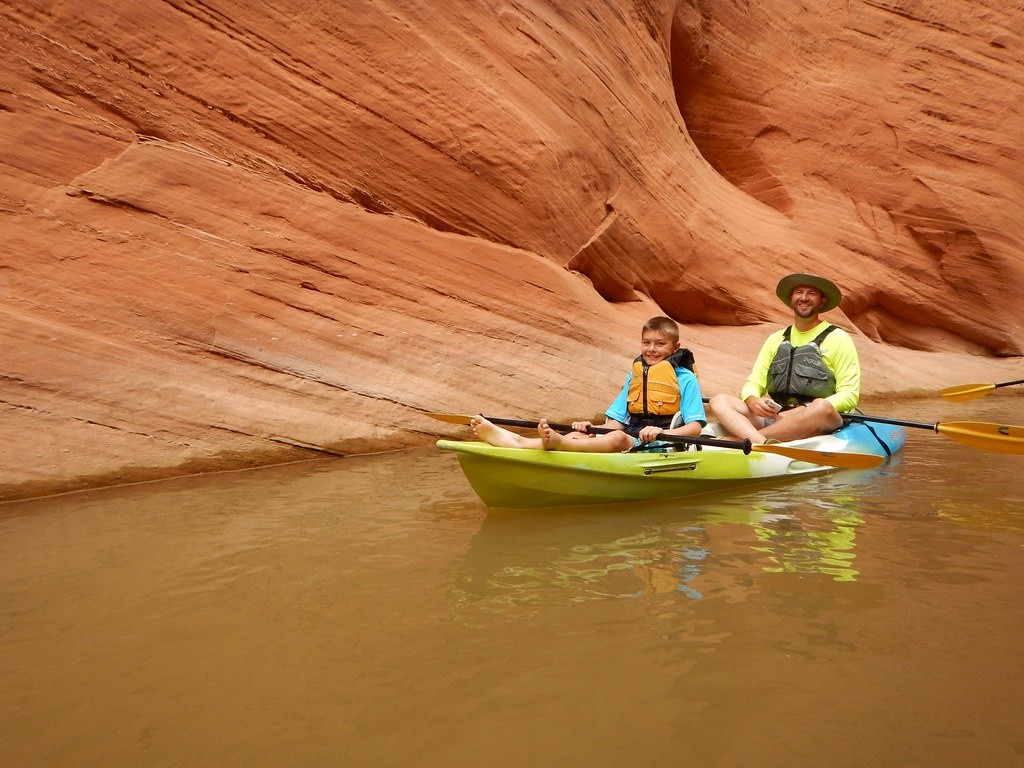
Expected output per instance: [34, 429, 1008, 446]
[421, 411, 886, 470]
[935, 379, 1024, 403]
[702, 397, 1024, 457]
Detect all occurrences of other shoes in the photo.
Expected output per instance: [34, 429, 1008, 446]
[709, 436, 723, 441]
[764, 438, 778, 444]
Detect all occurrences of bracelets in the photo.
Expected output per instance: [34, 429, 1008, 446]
[801, 403, 807, 408]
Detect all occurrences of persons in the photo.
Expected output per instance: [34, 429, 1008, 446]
[471, 316, 707, 453]
[709, 273, 861, 444]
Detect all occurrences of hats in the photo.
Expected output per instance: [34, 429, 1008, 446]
[776, 273, 842, 313]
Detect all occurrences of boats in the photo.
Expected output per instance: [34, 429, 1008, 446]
[433, 408, 903, 513]
